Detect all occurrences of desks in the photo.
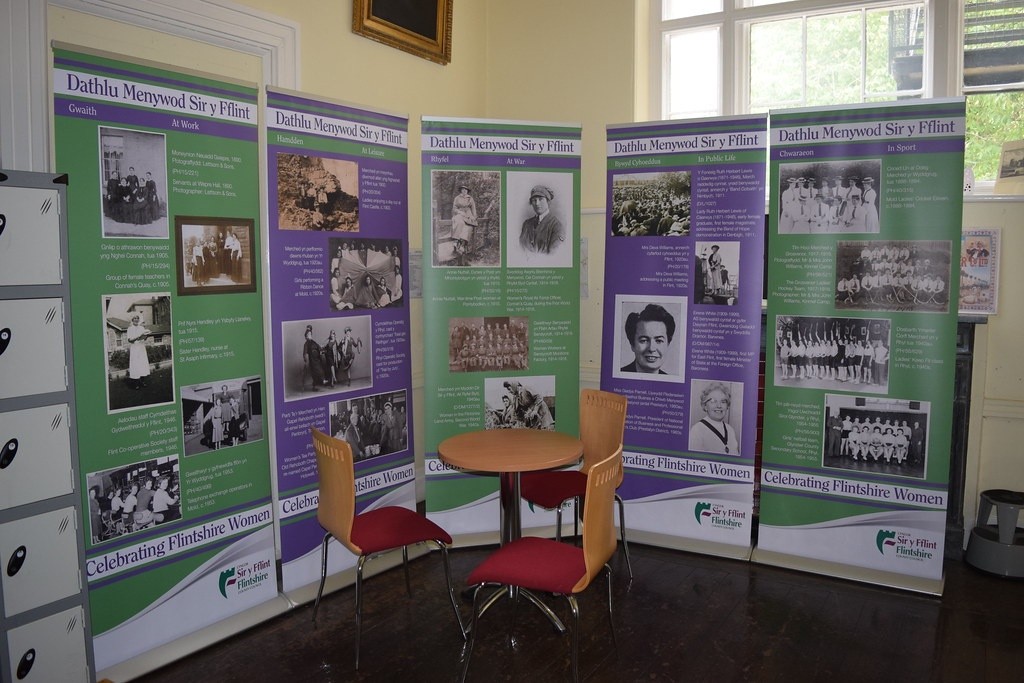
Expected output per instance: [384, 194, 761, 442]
[438, 427, 584, 648]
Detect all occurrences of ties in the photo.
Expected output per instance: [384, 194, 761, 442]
[838, 244, 946, 296]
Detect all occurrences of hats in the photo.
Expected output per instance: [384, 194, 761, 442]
[459, 183, 471, 191]
[841, 414, 920, 437]
[452, 321, 526, 349]
[530, 185, 554, 200]
[711, 245, 720, 252]
[787, 175, 875, 220]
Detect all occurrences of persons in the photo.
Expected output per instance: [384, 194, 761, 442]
[450, 184, 478, 255]
[777, 176, 990, 466]
[611, 184, 690, 236]
[620, 303, 676, 375]
[519, 185, 566, 256]
[331, 401, 407, 461]
[89, 475, 179, 544]
[104, 167, 160, 225]
[329, 240, 403, 311]
[304, 324, 363, 392]
[689, 383, 739, 455]
[127, 314, 150, 389]
[487, 380, 555, 430]
[448, 320, 529, 371]
[299, 181, 329, 215]
[211, 384, 241, 450]
[708, 244, 729, 296]
[182, 228, 244, 285]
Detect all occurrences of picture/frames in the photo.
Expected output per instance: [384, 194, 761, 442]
[957, 228, 1001, 315]
[352, 0, 453, 66]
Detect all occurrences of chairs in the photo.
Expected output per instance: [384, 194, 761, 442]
[510, 390, 634, 593]
[461, 443, 623, 683]
[310, 426, 466, 669]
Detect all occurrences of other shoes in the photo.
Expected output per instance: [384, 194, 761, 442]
[841, 452, 907, 467]
[779, 375, 886, 388]
